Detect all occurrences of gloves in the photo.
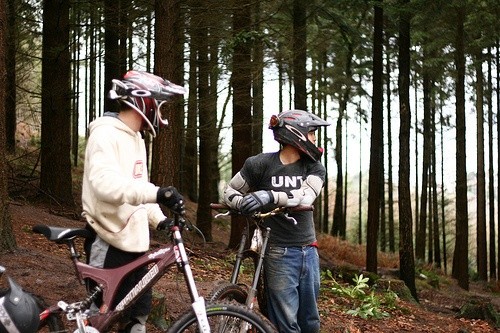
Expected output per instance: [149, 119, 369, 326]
[239, 190, 278, 212]
[156, 217, 186, 237]
[157, 186, 186, 216]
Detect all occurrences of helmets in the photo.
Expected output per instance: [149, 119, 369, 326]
[0, 265, 41, 333]
[267, 109, 332, 162]
[107, 70, 187, 131]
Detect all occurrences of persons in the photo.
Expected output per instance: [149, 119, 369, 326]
[80, 70, 185, 333]
[226, 110, 328, 333]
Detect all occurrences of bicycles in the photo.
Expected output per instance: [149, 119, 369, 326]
[203, 200, 315, 333]
[0, 184, 277, 333]
